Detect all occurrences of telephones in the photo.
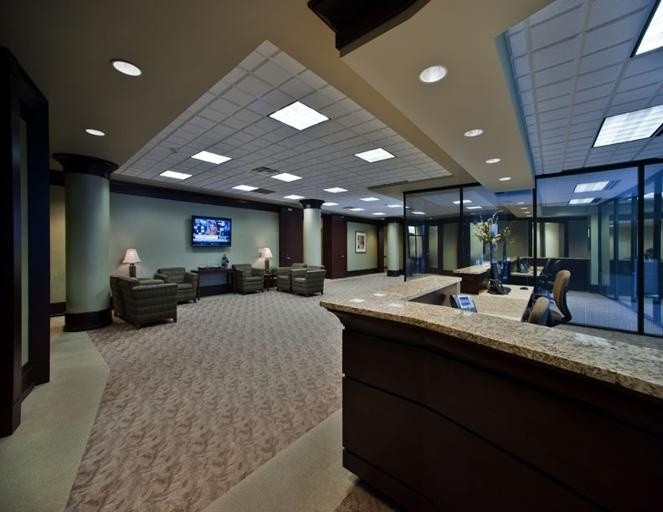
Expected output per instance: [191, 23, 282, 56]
[449, 293, 477, 312]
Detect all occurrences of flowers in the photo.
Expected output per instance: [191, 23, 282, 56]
[470, 210, 512, 242]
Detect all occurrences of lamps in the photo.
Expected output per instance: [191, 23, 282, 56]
[121, 249, 142, 277]
[259, 248, 273, 271]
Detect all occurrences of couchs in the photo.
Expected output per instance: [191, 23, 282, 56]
[276, 263, 306, 293]
[110, 276, 178, 330]
[291, 265, 327, 297]
[232, 264, 264, 294]
[154, 268, 198, 303]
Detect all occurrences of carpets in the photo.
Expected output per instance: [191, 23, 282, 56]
[63, 274, 434, 512]
[547, 291, 663, 339]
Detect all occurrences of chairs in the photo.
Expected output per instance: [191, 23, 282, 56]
[529, 296, 549, 325]
[539, 260, 566, 292]
[537, 275, 554, 303]
[547, 270, 572, 327]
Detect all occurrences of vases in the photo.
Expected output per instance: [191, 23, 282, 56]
[485, 241, 490, 260]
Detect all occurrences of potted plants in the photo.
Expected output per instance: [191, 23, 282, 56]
[269, 266, 277, 273]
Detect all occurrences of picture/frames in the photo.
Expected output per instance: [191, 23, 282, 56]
[355, 231, 367, 252]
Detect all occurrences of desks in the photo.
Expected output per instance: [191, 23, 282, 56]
[191, 268, 236, 300]
[461, 284, 534, 322]
[510, 266, 544, 293]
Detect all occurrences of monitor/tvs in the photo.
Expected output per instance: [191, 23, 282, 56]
[307, 1, 432, 57]
[190, 215, 233, 247]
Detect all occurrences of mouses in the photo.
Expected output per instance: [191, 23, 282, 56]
[520, 287, 529, 291]
